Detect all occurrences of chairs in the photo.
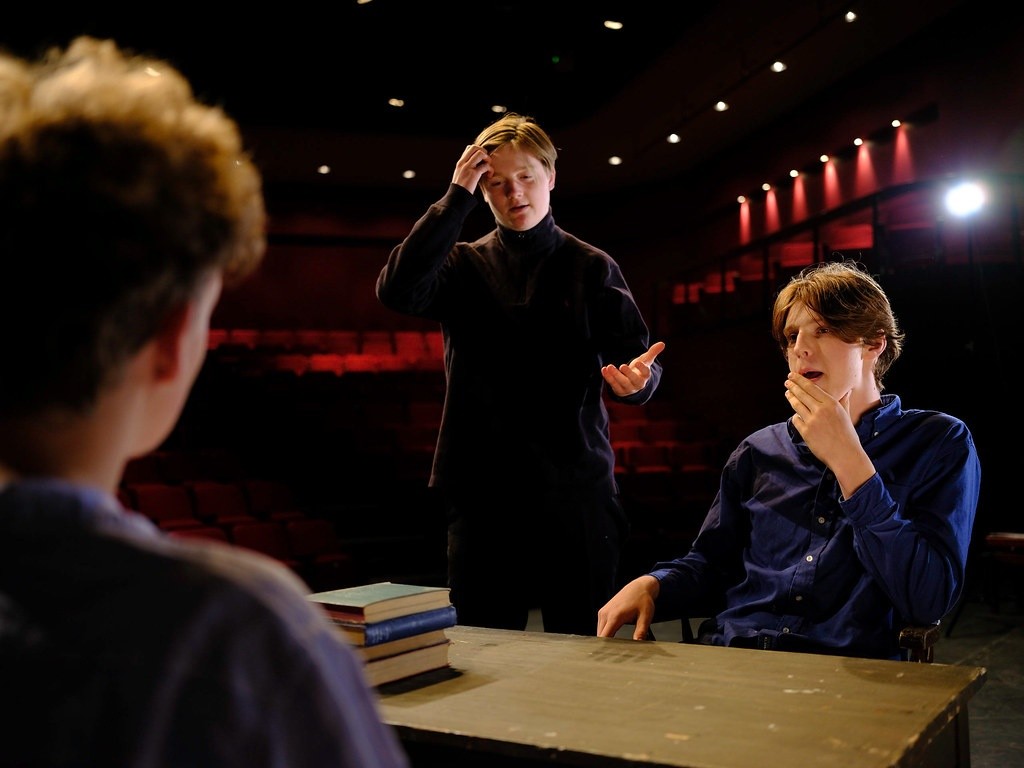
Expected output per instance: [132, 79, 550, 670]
[945, 530, 1024, 637]
[116, 328, 727, 583]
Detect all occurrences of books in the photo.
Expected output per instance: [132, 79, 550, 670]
[357, 641, 456, 687]
[344, 630, 449, 663]
[335, 607, 457, 647]
[312, 582, 454, 624]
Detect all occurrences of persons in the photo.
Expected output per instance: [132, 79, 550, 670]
[0, 38, 411, 768]
[377, 115, 665, 635]
[597, 264, 980, 664]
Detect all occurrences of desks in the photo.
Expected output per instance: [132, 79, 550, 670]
[375, 625, 988, 768]
